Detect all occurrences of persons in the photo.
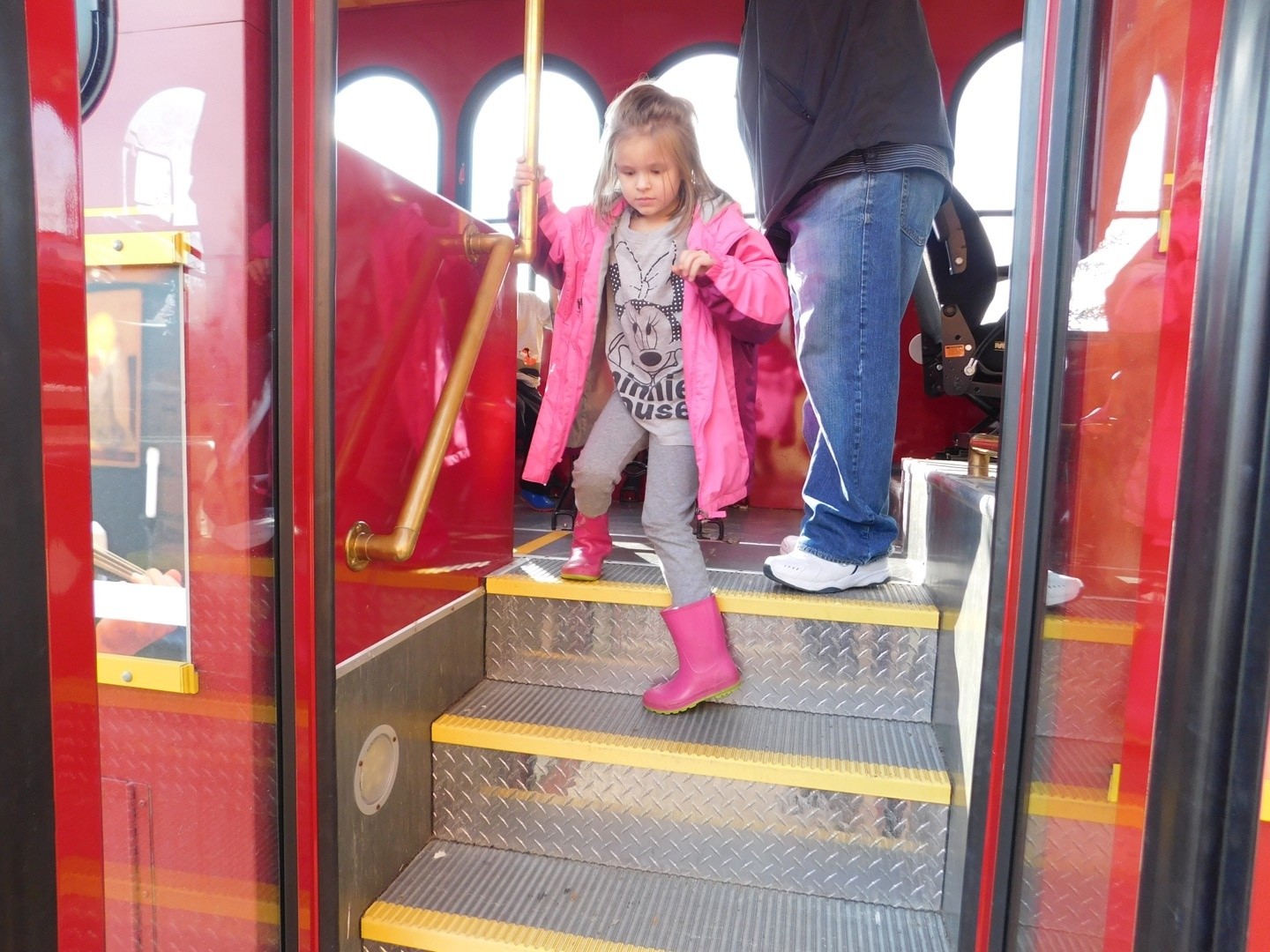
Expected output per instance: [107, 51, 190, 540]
[736, 2, 957, 593]
[514, 366, 565, 515]
[506, 84, 790, 714]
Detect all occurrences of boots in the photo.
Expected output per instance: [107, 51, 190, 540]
[559, 508, 616, 583]
[640, 592, 742, 716]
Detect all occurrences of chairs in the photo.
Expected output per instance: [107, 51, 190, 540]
[915, 173, 1008, 455]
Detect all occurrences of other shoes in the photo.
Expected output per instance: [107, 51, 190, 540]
[520, 488, 556, 512]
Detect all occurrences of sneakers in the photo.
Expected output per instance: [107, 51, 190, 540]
[1045, 568, 1084, 609]
[762, 533, 891, 594]
